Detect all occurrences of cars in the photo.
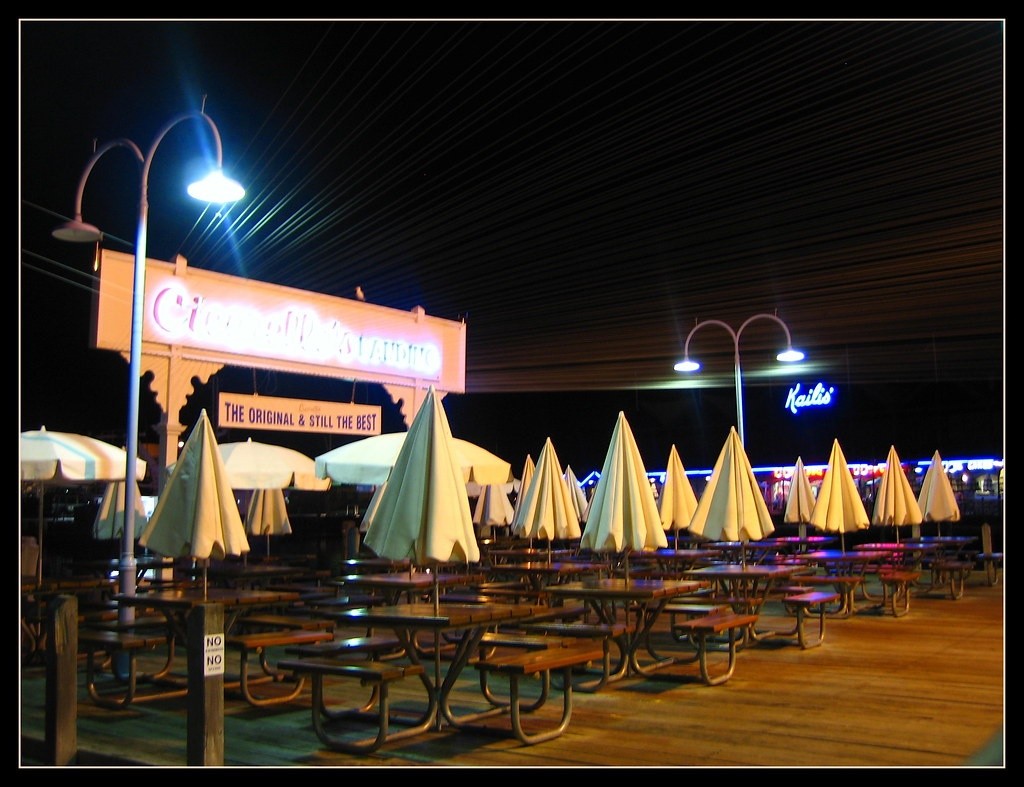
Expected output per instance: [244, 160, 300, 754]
[20, 490, 100, 549]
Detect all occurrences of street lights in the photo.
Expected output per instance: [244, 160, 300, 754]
[673, 313, 805, 447]
[51, 105, 248, 688]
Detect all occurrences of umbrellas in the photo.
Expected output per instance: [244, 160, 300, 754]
[689, 427, 775, 615]
[139, 406, 250, 599]
[21, 426, 148, 666]
[581, 410, 668, 677]
[872, 446, 923, 566]
[810, 438, 870, 577]
[781, 456, 817, 552]
[316, 431, 513, 574]
[466, 437, 699, 565]
[93, 434, 333, 567]
[918, 449, 961, 538]
[318, 381, 513, 732]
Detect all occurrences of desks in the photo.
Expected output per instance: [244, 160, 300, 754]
[48, 533, 978, 747]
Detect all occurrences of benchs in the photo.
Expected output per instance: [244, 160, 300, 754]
[22, 558, 1005, 746]
